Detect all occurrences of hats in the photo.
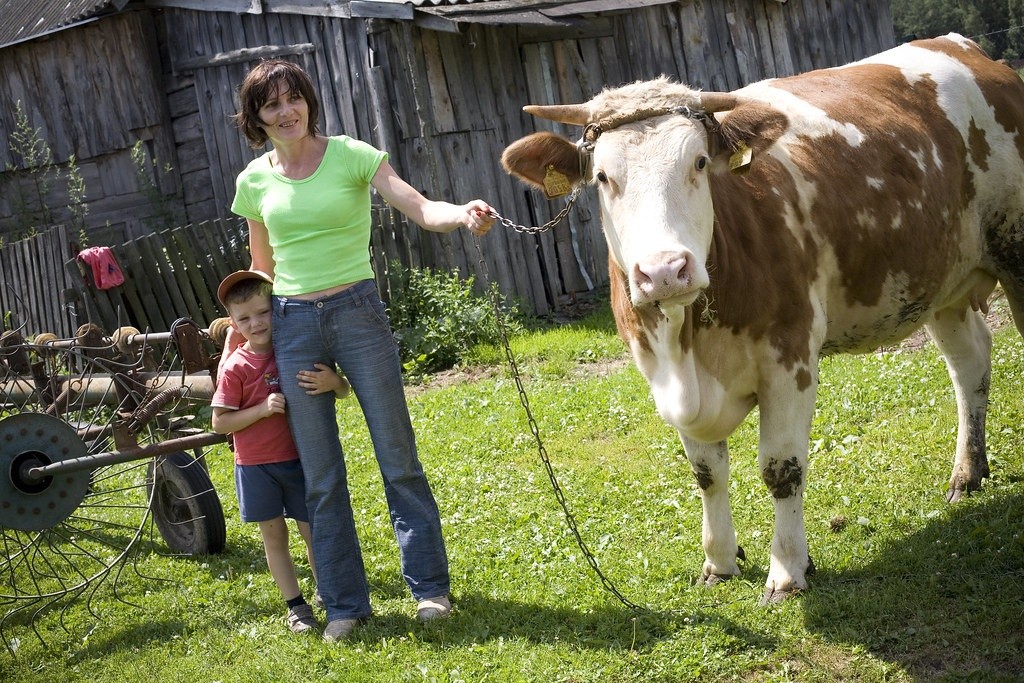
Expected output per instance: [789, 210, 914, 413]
[218, 270, 273, 307]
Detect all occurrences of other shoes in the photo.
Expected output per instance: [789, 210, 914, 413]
[417, 596, 451, 623]
[287, 604, 317, 634]
[321, 617, 358, 645]
[312, 589, 326, 608]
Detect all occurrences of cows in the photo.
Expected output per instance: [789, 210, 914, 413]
[496, 34, 1023, 609]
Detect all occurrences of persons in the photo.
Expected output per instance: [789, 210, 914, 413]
[216, 59, 496, 645]
[210, 270, 350, 632]
